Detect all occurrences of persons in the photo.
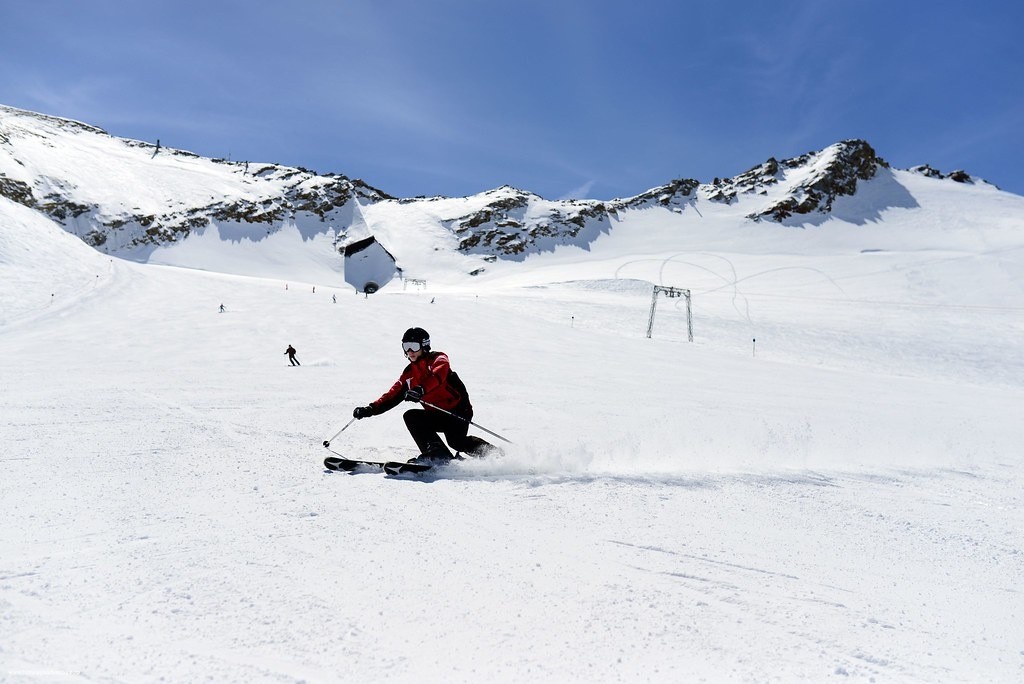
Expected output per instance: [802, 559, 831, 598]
[220, 304, 225, 312]
[353, 327, 505, 466]
[284, 344, 301, 366]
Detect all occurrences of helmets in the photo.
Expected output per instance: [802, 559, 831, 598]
[401, 326, 431, 358]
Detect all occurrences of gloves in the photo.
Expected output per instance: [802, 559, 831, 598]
[404, 384, 426, 403]
[353, 405, 374, 420]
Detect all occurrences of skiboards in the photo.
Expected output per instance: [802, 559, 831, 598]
[323, 456, 435, 480]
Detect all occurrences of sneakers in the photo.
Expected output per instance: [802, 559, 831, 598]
[408, 443, 451, 463]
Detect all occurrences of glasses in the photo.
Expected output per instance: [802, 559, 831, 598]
[403, 342, 423, 352]
